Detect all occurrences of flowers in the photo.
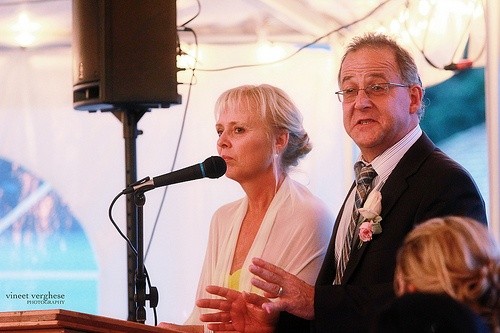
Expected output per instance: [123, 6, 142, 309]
[357, 215, 384, 242]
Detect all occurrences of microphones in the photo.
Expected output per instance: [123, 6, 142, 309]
[123, 156, 227, 194]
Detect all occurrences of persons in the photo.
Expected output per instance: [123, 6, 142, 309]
[375, 292, 480, 333]
[394, 216, 500, 333]
[157, 84, 335, 333]
[196, 33, 488, 333]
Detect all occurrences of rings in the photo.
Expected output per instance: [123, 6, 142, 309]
[277, 286, 283, 297]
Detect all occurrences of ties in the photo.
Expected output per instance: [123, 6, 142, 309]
[333, 162, 378, 285]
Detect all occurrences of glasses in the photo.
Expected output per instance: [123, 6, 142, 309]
[334, 82, 410, 103]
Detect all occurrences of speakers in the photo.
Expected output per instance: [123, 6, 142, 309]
[72, 0, 182, 113]
